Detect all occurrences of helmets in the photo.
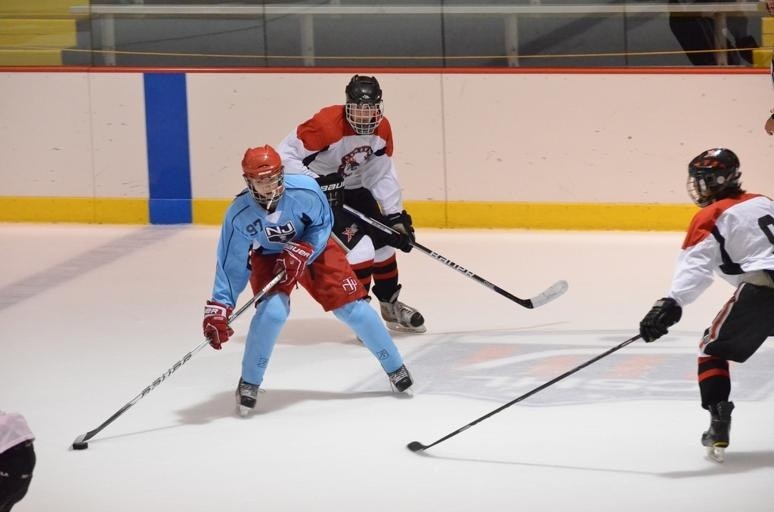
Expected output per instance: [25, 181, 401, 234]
[345, 75, 384, 135]
[687, 148, 742, 207]
[241, 144, 285, 205]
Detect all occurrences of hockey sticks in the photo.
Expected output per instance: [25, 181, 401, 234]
[72, 266, 302, 450]
[339, 198, 568, 308]
[406, 330, 662, 453]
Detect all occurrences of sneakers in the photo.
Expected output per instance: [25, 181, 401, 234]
[387, 364, 412, 392]
[239, 377, 259, 408]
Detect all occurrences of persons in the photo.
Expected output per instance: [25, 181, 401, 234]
[666, 0, 761, 66]
[638, 147, 772, 460]
[279, 75, 426, 334]
[200, 143, 414, 411]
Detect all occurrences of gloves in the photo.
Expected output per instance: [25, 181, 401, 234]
[272, 241, 314, 288]
[314, 173, 347, 221]
[639, 298, 682, 342]
[204, 300, 235, 350]
[386, 209, 415, 252]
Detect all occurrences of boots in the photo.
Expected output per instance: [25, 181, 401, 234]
[701, 399, 734, 448]
[372, 284, 423, 327]
